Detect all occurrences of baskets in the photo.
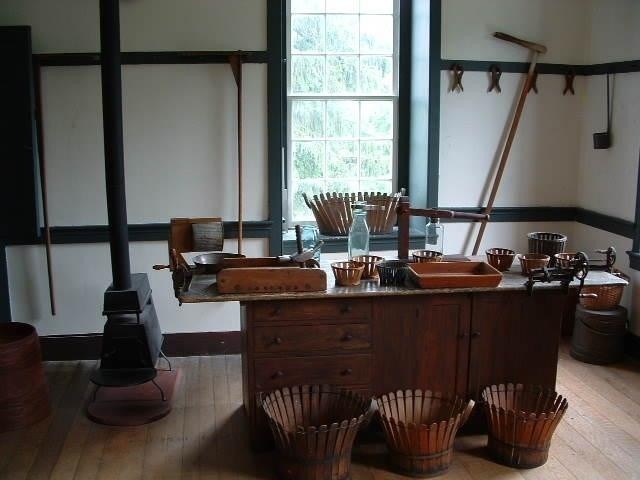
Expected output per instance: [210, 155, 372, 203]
[577, 283, 625, 310]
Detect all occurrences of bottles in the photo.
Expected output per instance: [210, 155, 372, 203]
[425, 216, 444, 259]
[347, 200, 371, 263]
[300, 225, 321, 268]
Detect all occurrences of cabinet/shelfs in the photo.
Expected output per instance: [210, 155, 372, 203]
[241, 290, 568, 452]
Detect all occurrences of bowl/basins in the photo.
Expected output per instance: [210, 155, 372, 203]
[193, 251, 247, 274]
